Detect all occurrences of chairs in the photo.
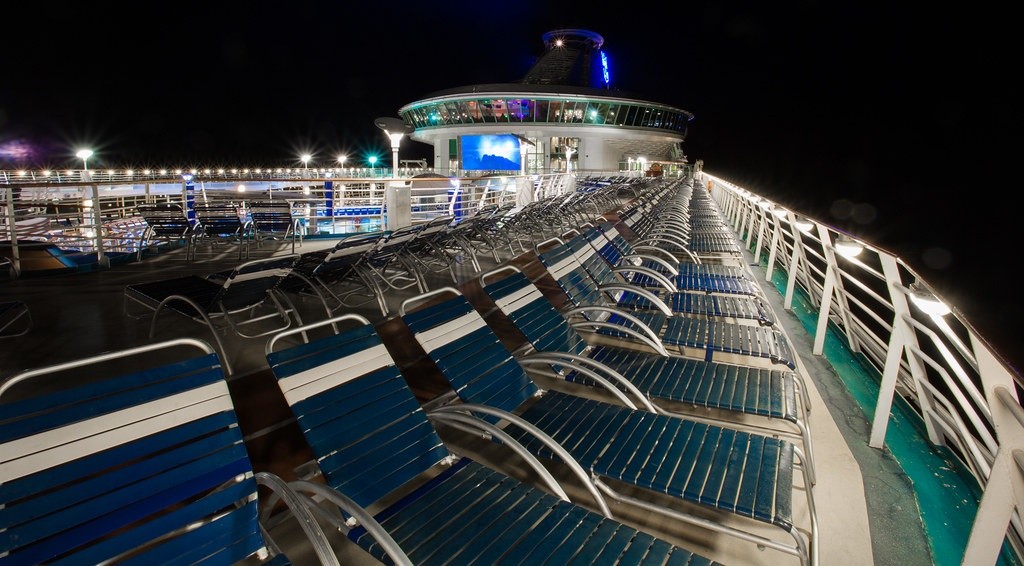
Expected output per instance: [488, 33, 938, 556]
[0, 174, 822, 566]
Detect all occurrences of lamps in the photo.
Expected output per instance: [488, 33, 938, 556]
[759, 197, 769, 208]
[748, 193, 758, 201]
[773, 205, 787, 217]
[834, 234, 863, 256]
[563, 144, 577, 173]
[793, 216, 814, 231]
[910, 276, 951, 317]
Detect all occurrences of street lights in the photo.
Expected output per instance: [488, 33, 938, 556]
[375, 133, 415, 178]
[338, 155, 347, 168]
[302, 153, 310, 168]
[76, 148, 94, 169]
[370, 155, 377, 167]
